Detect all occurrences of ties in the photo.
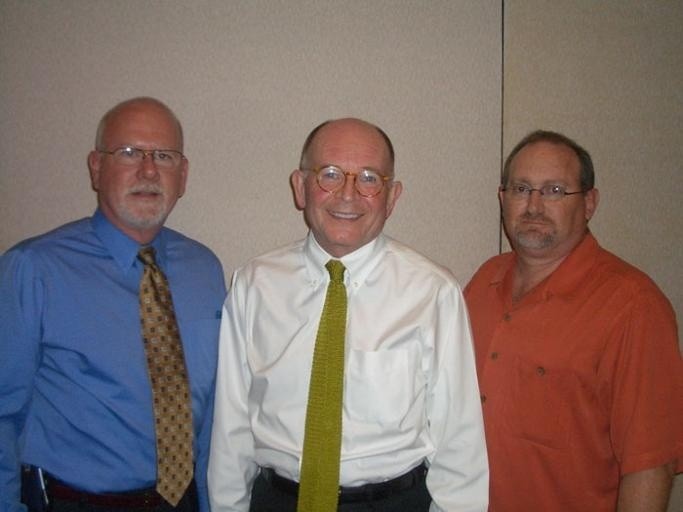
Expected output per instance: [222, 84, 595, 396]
[296, 259, 346, 512]
[138, 246, 195, 507]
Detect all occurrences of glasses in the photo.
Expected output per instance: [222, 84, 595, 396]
[99, 148, 185, 168]
[502, 182, 588, 201]
[301, 164, 395, 197]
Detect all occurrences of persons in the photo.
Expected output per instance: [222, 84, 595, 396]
[0, 97, 225, 512]
[460, 128, 682, 511]
[204, 117, 491, 511]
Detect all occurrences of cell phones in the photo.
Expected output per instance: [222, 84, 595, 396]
[21, 465, 50, 512]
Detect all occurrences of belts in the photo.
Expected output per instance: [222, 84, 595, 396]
[49, 479, 198, 505]
[260, 468, 423, 501]
